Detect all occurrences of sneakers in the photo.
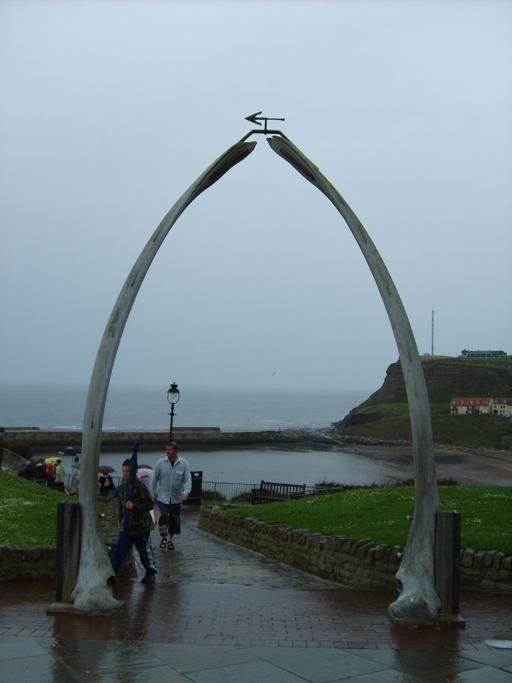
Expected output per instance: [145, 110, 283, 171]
[140, 573, 157, 585]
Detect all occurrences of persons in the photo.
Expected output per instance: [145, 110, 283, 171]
[19, 443, 152, 494]
[99, 460, 159, 585]
[145, 441, 192, 551]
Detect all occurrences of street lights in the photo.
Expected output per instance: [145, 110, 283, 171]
[166, 383, 181, 443]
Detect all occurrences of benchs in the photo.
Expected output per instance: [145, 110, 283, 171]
[251, 478, 304, 504]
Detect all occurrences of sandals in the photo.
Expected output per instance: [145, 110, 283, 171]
[158, 538, 168, 549]
[167, 540, 175, 551]
